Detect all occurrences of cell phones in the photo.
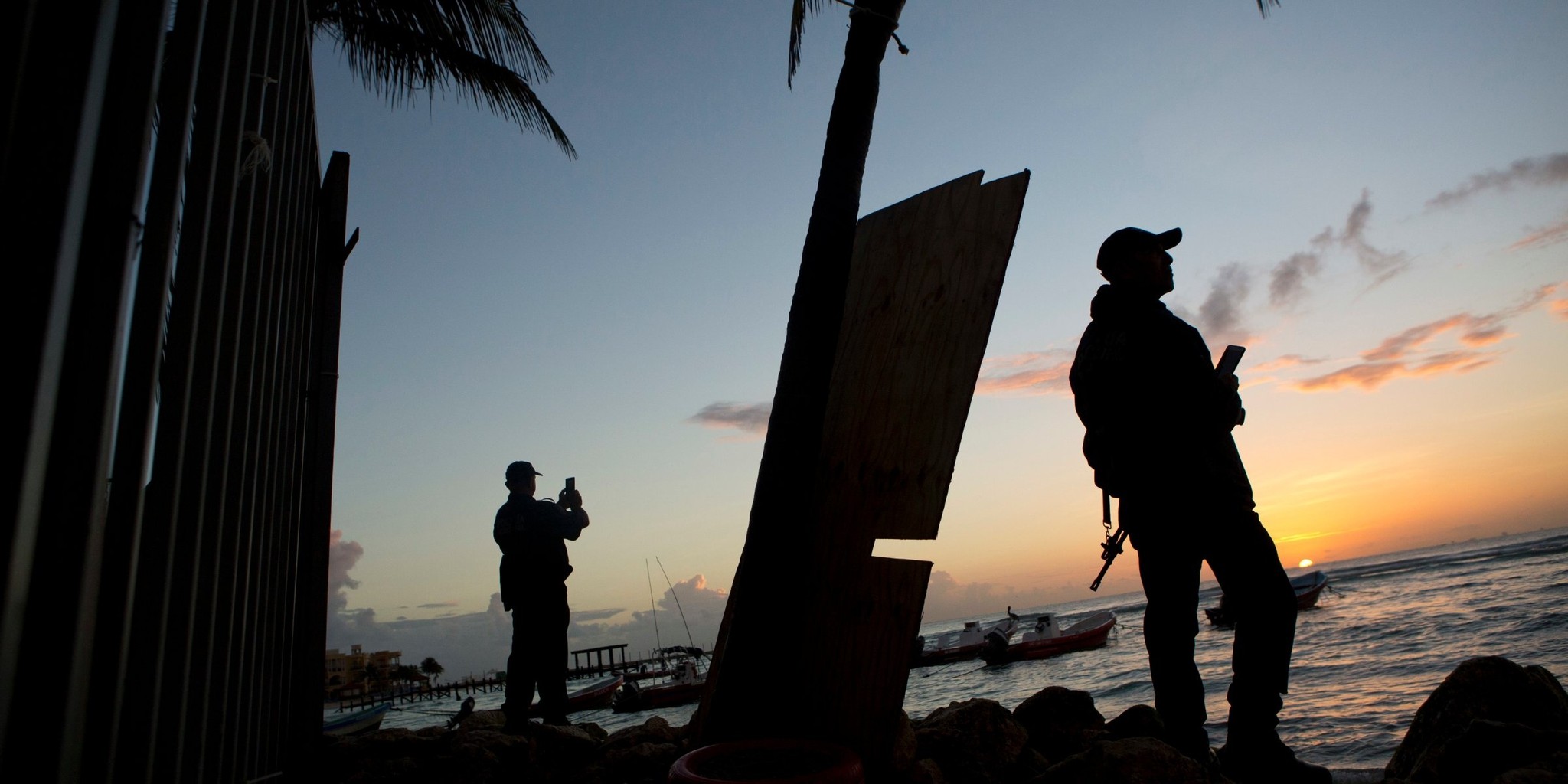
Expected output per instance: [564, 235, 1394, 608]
[1215, 345, 1246, 380]
[566, 477, 575, 498]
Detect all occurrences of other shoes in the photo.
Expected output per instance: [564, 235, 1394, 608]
[544, 717, 572, 726]
[518, 720, 541, 735]
[1191, 747, 1223, 781]
[1282, 758, 1333, 783]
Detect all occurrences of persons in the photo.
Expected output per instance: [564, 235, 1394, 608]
[1069, 226, 1336, 784]
[494, 461, 590, 737]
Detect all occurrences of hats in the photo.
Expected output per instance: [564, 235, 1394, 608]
[1098, 228, 1184, 274]
[507, 462, 543, 480]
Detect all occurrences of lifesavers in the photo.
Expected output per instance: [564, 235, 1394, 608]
[667, 739, 864, 784]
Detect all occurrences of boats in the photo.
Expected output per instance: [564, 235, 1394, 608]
[611, 669, 676, 682]
[975, 643, 1020, 665]
[1013, 613, 1117, 661]
[528, 673, 622, 719]
[609, 661, 708, 714]
[323, 702, 391, 738]
[916, 620, 1019, 667]
[1203, 570, 1329, 628]
